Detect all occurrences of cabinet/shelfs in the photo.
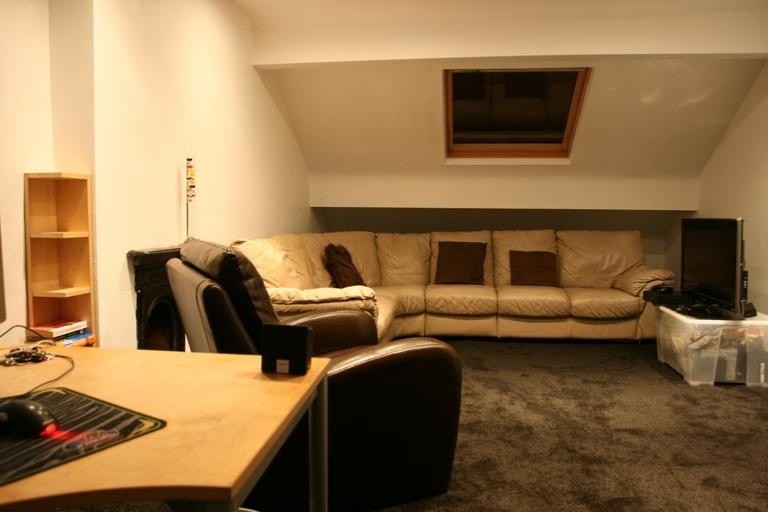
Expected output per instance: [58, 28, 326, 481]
[22, 173, 96, 345]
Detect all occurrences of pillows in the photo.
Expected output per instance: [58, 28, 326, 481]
[323, 240, 559, 288]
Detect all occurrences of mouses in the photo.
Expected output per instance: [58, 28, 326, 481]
[1, 398, 59, 440]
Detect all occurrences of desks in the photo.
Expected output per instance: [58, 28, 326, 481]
[0, 347, 332, 511]
[660, 305, 768, 388]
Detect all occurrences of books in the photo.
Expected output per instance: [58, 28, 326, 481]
[54, 331, 97, 347]
[26, 328, 85, 341]
[31, 319, 88, 338]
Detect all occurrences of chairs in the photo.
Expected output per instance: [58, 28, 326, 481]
[168, 237, 461, 512]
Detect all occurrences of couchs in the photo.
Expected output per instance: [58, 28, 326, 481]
[237, 229, 675, 340]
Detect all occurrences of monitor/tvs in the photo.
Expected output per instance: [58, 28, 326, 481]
[680, 217, 757, 321]
